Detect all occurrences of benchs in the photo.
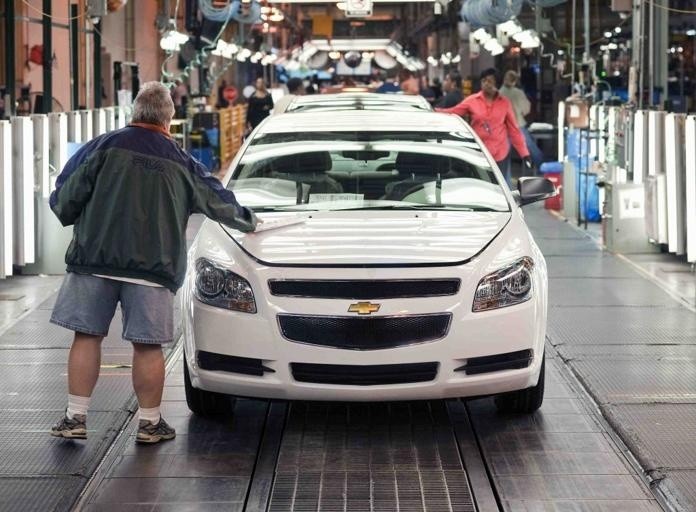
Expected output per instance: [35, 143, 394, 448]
[326, 170, 396, 200]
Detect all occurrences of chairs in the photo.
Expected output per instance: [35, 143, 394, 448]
[272, 151, 345, 193]
[390, 151, 457, 202]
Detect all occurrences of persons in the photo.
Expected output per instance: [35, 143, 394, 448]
[498, 70, 554, 190]
[434, 66, 530, 190]
[172, 71, 468, 133]
[48, 78, 262, 448]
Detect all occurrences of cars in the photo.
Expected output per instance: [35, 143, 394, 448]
[180, 93, 557, 415]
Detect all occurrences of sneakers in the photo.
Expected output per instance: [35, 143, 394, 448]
[134, 413, 176, 443]
[50, 408, 88, 439]
